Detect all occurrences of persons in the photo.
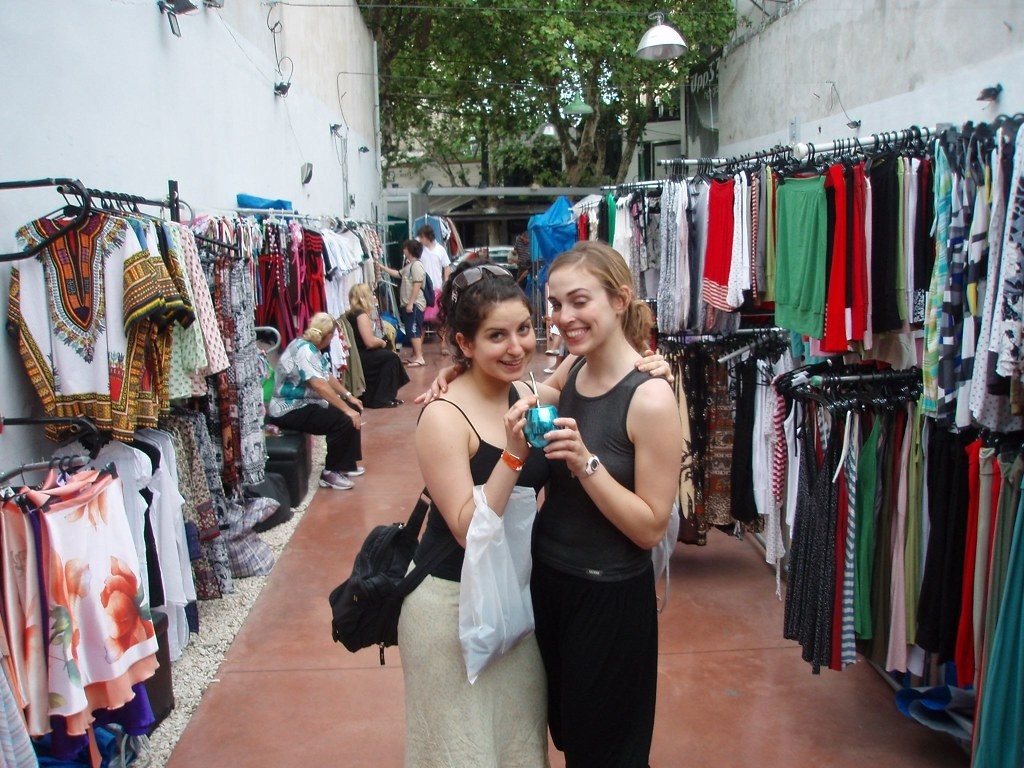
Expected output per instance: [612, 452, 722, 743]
[377, 239, 427, 367]
[346, 283, 410, 408]
[511, 231, 531, 291]
[268, 309, 365, 489]
[413, 240, 681, 768]
[396, 259, 676, 768]
[516, 261, 544, 286]
[416, 224, 452, 355]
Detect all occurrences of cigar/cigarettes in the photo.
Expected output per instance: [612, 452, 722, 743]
[361, 423, 366, 425]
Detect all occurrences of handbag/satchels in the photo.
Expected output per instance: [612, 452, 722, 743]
[329, 522, 419, 666]
[652, 501, 680, 614]
[459, 483, 538, 684]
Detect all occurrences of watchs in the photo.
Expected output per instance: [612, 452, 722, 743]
[571, 453, 600, 480]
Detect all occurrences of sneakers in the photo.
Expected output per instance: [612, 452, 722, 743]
[318, 470, 355, 490]
[339, 466, 365, 477]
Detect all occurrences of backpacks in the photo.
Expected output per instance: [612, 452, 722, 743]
[410, 263, 434, 307]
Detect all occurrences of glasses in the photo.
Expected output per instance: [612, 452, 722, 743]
[451, 265, 513, 309]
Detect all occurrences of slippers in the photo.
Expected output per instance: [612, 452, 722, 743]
[407, 362, 426, 367]
[402, 359, 412, 364]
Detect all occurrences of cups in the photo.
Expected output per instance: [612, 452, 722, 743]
[522, 405, 559, 448]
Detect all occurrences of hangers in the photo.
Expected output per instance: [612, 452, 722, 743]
[4, 393, 200, 503]
[579, 112, 1024, 208]
[17, 182, 383, 268]
[639, 327, 1024, 459]
[423, 209, 449, 221]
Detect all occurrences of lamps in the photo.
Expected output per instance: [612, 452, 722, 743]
[633, 11, 687, 61]
[421, 180, 434, 194]
[561, 89, 595, 114]
[534, 116, 558, 136]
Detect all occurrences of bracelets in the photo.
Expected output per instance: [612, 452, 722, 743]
[500, 449, 525, 472]
[345, 392, 351, 397]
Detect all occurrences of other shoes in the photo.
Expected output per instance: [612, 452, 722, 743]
[383, 398, 405, 408]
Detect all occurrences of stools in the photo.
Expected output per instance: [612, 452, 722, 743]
[262, 423, 311, 503]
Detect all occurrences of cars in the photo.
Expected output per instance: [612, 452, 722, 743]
[451, 248, 519, 275]
